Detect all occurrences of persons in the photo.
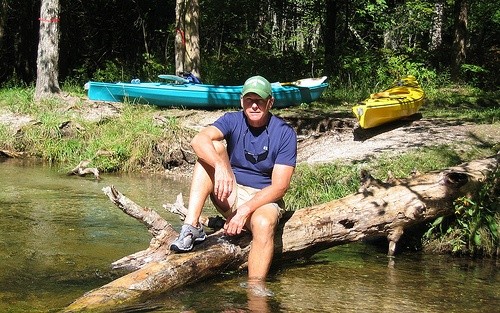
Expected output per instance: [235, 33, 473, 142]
[170, 76, 297, 279]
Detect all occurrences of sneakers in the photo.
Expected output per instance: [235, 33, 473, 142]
[170, 223, 206, 252]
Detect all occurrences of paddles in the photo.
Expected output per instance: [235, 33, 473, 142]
[158, 75, 201, 84]
[280, 75, 327, 86]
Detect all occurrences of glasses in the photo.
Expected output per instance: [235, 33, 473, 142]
[243, 126, 269, 156]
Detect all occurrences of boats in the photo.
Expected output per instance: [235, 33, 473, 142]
[352, 74, 425, 130]
[85, 81, 329, 111]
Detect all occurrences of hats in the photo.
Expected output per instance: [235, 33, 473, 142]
[243, 76, 272, 99]
